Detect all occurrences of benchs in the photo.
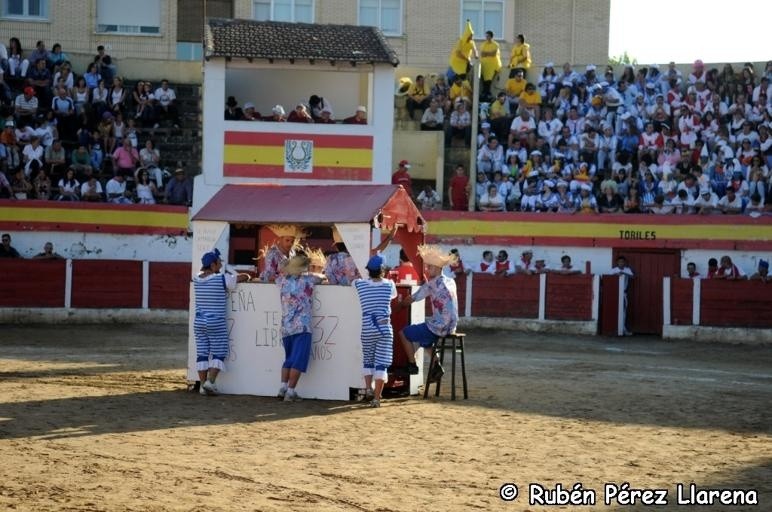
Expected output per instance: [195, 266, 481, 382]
[395, 91, 480, 209]
[1, 64, 201, 203]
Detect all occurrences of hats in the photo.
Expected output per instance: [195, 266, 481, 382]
[200, 252, 218, 270]
[226, 95, 367, 116]
[401, 160, 410, 168]
[368, 256, 386, 269]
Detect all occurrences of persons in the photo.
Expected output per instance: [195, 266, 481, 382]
[350, 255, 399, 409]
[275, 252, 327, 404]
[192, 247, 252, 397]
[392, 17, 772, 216]
[683, 255, 772, 285]
[306, 247, 327, 273]
[448, 247, 466, 275]
[393, 242, 459, 383]
[385, 247, 422, 287]
[324, 229, 363, 286]
[258, 224, 314, 284]
[1, 233, 22, 259]
[608, 256, 634, 338]
[476, 248, 581, 276]
[32, 241, 64, 259]
[223, 89, 370, 128]
[1, 36, 192, 208]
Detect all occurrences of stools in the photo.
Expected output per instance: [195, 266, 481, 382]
[421, 331, 469, 401]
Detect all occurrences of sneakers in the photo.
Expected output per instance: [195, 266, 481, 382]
[278, 388, 304, 402]
[199, 380, 220, 395]
[367, 389, 381, 407]
[407, 366, 419, 374]
[428, 365, 444, 383]
